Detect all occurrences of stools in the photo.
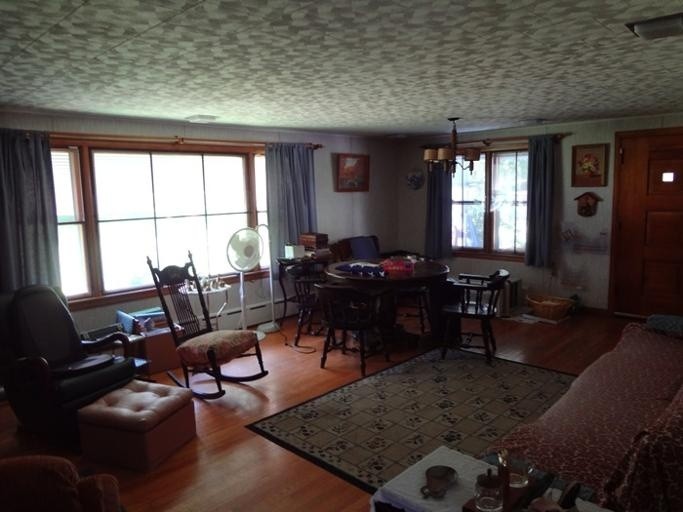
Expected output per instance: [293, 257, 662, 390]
[77, 378, 197, 474]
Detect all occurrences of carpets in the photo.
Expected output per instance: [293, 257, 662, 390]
[243, 346, 580, 495]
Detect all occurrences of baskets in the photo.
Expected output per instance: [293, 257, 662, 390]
[527, 295, 575, 321]
[300, 232, 328, 250]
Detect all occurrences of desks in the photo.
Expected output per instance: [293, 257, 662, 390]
[366, 445, 617, 512]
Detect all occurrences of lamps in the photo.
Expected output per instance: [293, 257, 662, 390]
[624, 12, 683, 42]
[423, 117, 480, 178]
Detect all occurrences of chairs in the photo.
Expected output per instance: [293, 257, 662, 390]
[278, 260, 511, 376]
[145, 250, 268, 400]
[0, 285, 151, 447]
[331, 235, 410, 260]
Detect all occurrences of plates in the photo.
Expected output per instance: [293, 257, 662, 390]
[446, 467, 458, 486]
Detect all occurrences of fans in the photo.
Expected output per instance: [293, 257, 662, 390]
[226, 226, 266, 343]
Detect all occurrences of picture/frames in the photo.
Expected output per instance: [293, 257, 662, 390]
[571, 142, 607, 188]
[336, 153, 369, 192]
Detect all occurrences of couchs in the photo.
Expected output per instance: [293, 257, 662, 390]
[486, 314, 682, 512]
[0, 453, 128, 512]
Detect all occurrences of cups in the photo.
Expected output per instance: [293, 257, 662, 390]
[508, 456, 535, 488]
[421, 466, 451, 500]
[474, 474, 503, 511]
